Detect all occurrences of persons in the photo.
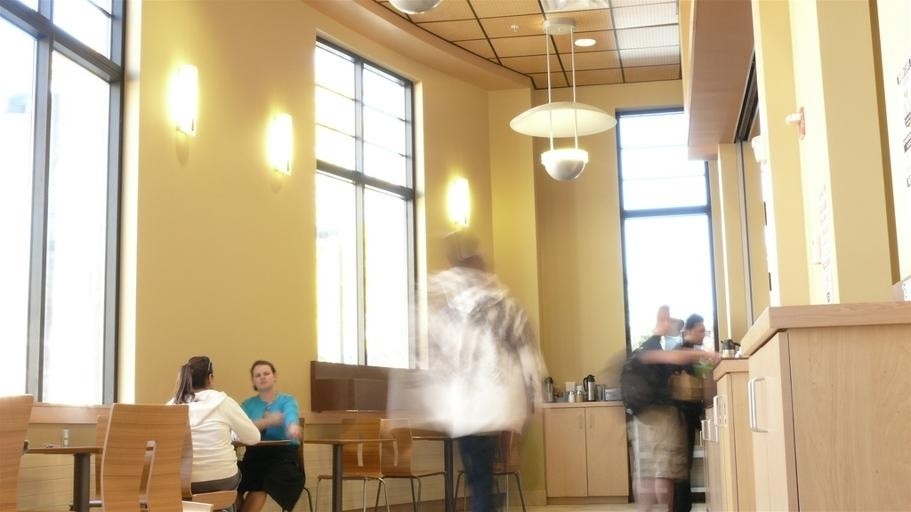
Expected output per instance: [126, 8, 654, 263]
[234, 361, 306, 512]
[628, 305, 722, 512]
[410, 229, 541, 510]
[164, 355, 262, 493]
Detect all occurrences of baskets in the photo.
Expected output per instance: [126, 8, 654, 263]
[670, 370, 704, 402]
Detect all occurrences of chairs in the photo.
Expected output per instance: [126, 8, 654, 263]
[178, 412, 238, 511]
[315, 413, 392, 511]
[291, 417, 312, 511]
[1, 394, 28, 511]
[453, 420, 528, 512]
[99, 402, 190, 511]
[375, 417, 452, 511]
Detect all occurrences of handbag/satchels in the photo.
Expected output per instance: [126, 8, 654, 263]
[620, 372, 658, 414]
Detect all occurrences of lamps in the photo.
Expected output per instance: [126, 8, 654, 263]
[509, 19, 617, 183]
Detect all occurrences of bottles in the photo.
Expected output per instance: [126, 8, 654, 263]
[568, 390, 583, 403]
[545, 377, 554, 402]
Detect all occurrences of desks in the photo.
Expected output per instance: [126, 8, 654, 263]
[23, 445, 104, 510]
[410, 435, 501, 510]
[303, 437, 394, 510]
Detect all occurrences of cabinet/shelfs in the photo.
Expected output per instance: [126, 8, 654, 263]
[544, 407, 632, 504]
[699, 372, 754, 512]
[750, 326, 910, 511]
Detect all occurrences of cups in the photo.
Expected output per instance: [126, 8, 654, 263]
[582, 375, 595, 402]
